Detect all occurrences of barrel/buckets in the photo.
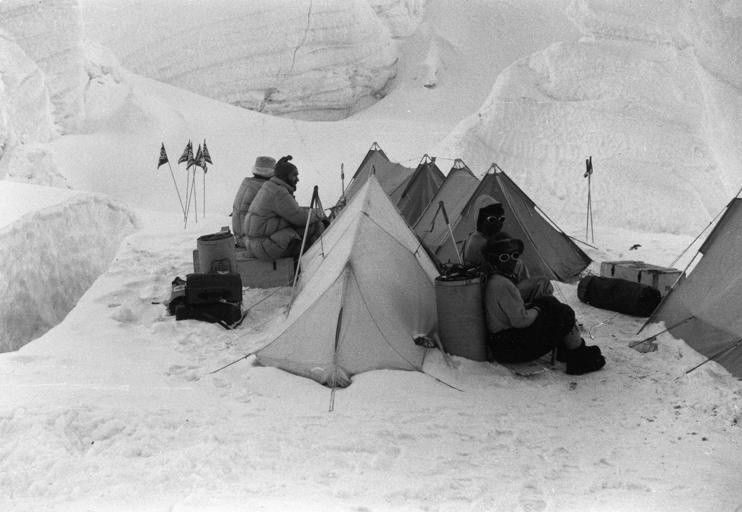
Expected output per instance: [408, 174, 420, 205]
[435, 276, 486, 361]
[197, 234, 238, 276]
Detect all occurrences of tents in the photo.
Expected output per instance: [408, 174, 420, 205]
[254, 139, 593, 389]
[647, 196, 741, 381]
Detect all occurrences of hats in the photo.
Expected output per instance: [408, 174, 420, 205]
[252, 156, 276, 177]
[276, 155, 296, 178]
[488, 232, 524, 254]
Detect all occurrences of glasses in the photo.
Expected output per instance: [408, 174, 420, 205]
[486, 215, 507, 225]
[499, 251, 520, 262]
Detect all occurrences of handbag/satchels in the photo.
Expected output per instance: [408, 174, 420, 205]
[576, 274, 662, 317]
[184, 273, 243, 326]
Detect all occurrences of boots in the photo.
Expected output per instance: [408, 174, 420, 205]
[554, 337, 607, 376]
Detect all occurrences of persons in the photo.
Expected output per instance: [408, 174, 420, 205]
[243, 154, 329, 276]
[232, 155, 275, 247]
